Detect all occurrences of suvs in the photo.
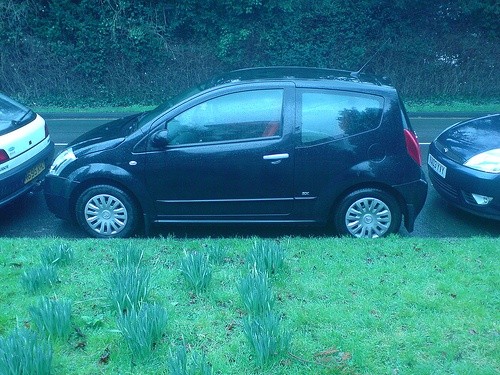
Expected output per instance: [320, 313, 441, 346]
[43, 65, 429, 238]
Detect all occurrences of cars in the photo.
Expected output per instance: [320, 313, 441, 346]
[427, 112, 500, 223]
[0, 92, 54, 209]
